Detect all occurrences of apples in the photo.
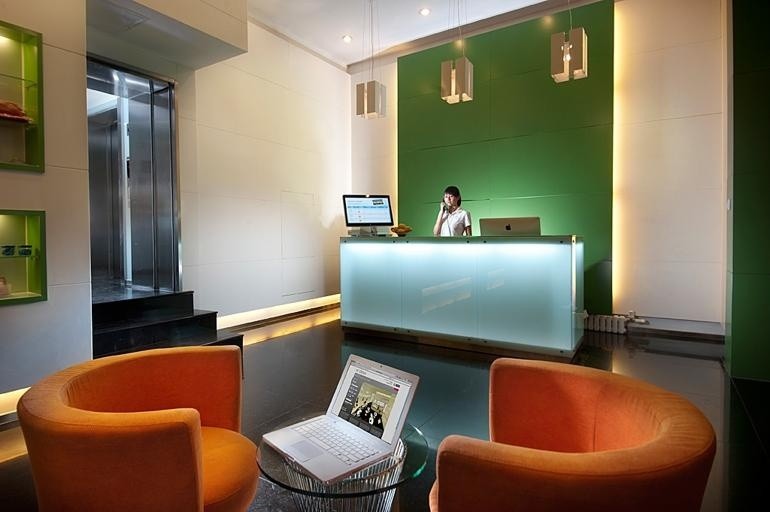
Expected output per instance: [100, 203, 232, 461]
[391, 223, 412, 231]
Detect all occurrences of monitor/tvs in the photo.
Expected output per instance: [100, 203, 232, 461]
[343, 195, 394, 237]
[480, 217, 540, 236]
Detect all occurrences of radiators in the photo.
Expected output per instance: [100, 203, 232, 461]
[583, 314, 627, 335]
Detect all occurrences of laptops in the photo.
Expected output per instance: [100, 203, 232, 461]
[262, 354, 420, 486]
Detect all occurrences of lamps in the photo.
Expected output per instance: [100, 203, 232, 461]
[550, 0, 587, 83]
[356, 0, 386, 118]
[442, 1, 472, 104]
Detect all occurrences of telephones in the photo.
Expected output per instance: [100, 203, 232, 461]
[441, 197, 449, 210]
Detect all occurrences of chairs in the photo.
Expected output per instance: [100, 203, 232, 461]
[16, 344, 260, 511]
[429, 358, 717, 512]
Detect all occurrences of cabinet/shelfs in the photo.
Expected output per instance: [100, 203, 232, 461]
[0, 21, 46, 305]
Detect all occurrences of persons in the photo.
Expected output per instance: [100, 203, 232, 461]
[433, 186, 472, 236]
